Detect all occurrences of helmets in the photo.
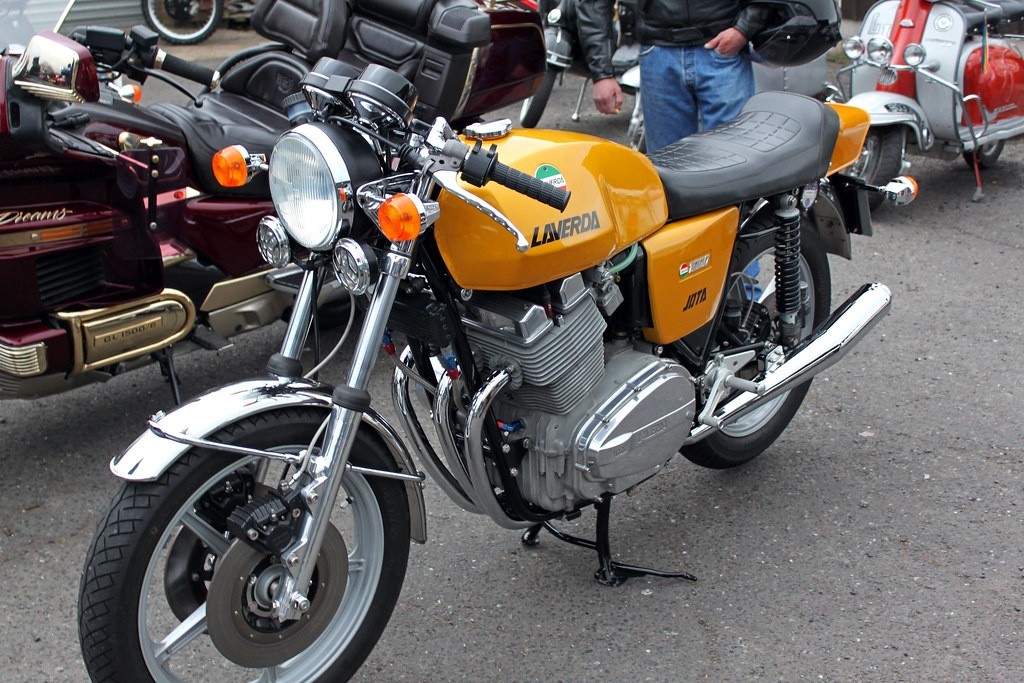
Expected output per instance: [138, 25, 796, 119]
[743, 0, 845, 70]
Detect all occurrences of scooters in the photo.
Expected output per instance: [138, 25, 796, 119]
[140, 0, 254, 45]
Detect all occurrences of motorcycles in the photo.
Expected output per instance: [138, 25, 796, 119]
[74, 53, 892, 683]
[0, 0, 544, 412]
[825, 0, 1024, 212]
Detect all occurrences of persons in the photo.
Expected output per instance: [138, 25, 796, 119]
[573, 0, 770, 152]
